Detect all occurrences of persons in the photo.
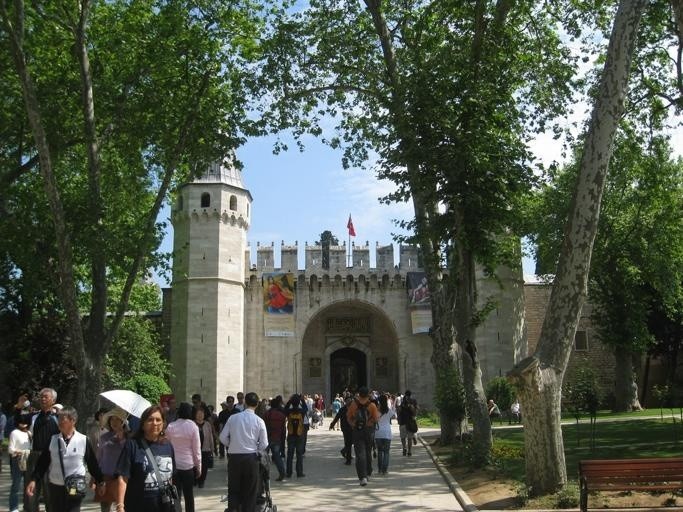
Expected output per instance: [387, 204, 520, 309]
[87, 405, 140, 511]
[266, 275, 292, 313]
[407, 275, 430, 306]
[510, 399, 521, 422]
[168, 391, 326, 511]
[328, 383, 423, 489]
[116, 406, 182, 511]
[485, 398, 500, 419]
[5, 387, 106, 510]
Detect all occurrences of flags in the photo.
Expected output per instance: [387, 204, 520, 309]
[346, 213, 355, 236]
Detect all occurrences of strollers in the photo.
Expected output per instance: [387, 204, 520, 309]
[224, 448, 279, 511]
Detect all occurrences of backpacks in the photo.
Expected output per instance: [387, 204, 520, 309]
[351, 398, 373, 438]
[287, 411, 305, 436]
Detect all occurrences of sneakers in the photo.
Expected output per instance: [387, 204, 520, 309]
[360, 475, 371, 486]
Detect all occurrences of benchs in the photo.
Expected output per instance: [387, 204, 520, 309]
[577, 459, 682, 511]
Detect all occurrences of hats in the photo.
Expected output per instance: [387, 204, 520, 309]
[359, 386, 368, 393]
[102, 408, 128, 431]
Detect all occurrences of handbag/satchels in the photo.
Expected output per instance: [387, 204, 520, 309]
[64, 473, 90, 497]
[158, 481, 182, 512]
[93, 476, 120, 502]
